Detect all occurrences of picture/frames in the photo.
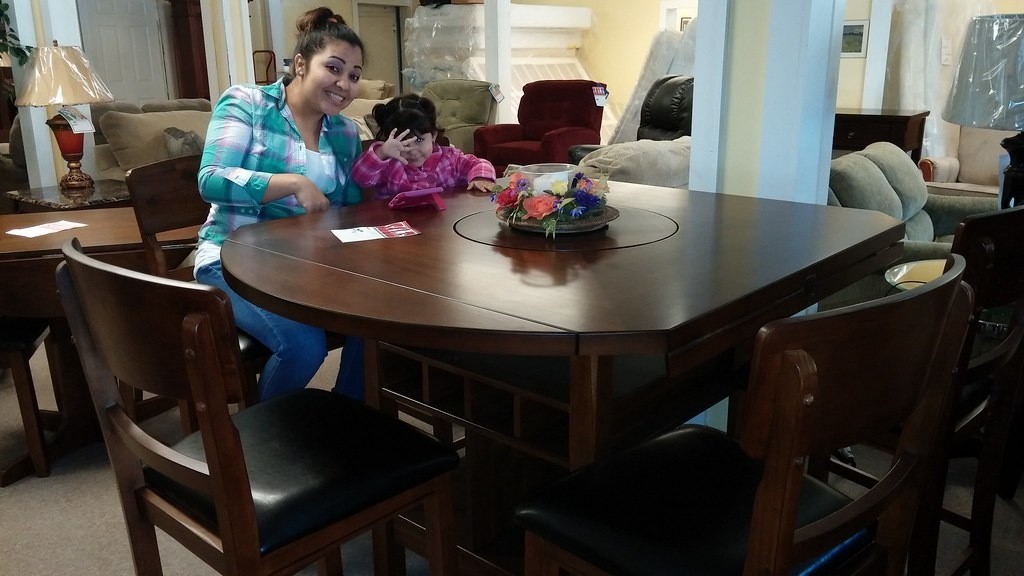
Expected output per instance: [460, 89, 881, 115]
[839, 19, 870, 58]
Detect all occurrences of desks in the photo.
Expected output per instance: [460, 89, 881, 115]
[831, 107, 930, 167]
[221, 181, 908, 576]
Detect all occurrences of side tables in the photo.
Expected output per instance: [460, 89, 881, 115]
[6, 179, 133, 214]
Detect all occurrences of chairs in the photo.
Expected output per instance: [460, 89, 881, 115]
[55, 237, 461, 576]
[125, 154, 346, 437]
[511, 253, 980, 576]
[807, 204, 1024, 576]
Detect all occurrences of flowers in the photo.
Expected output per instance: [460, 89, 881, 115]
[488, 170, 608, 241]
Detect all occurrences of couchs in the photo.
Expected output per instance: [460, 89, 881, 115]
[0, 99, 212, 188]
[472, 80, 608, 179]
[421, 78, 505, 155]
[338, 79, 396, 140]
[816, 142, 999, 312]
[567, 73, 694, 166]
[917, 125, 1021, 198]
[578, 135, 689, 190]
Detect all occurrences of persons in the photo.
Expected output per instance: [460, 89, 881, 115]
[351, 94, 497, 199]
[192, 6, 365, 400]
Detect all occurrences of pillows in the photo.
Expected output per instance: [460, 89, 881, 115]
[164, 126, 206, 158]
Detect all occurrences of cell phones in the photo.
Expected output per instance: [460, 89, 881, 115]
[388, 186, 444, 208]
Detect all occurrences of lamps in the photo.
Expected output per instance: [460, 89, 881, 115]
[939, 13, 1024, 209]
[14, 39, 114, 188]
